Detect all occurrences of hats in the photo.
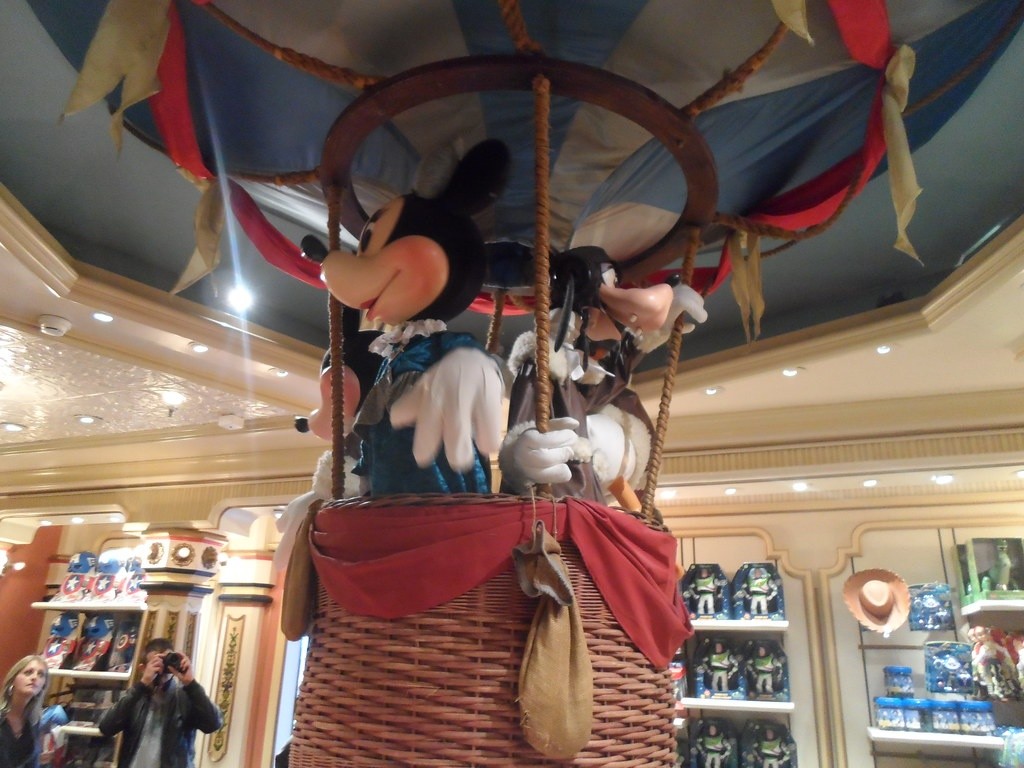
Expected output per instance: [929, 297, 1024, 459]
[844, 569, 912, 633]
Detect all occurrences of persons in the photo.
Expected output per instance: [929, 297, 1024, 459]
[969, 626, 1024, 701]
[689, 568, 722, 614]
[0, 654, 48, 768]
[753, 727, 790, 768]
[703, 641, 738, 691]
[100, 636, 224, 768]
[742, 567, 777, 615]
[747, 645, 783, 693]
[697, 724, 731, 768]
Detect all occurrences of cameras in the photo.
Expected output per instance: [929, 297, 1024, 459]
[158, 651, 183, 674]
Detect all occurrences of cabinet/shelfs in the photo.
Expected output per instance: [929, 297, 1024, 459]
[680, 619, 796, 714]
[31, 601, 148, 738]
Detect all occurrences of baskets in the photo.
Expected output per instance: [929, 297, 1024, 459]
[287, 494, 675, 768]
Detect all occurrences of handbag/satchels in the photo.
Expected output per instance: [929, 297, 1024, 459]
[518, 504, 591, 757]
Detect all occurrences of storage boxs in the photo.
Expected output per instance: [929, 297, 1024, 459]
[950, 537, 1024, 607]
